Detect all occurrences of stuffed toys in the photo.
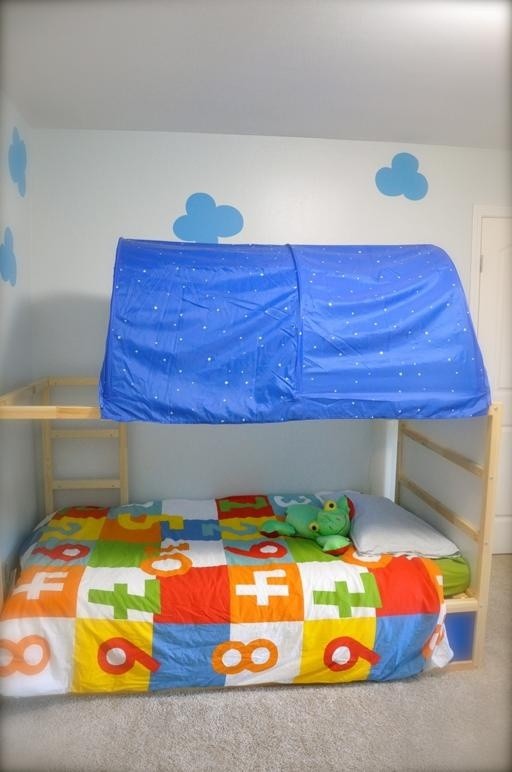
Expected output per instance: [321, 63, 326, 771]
[259, 494, 355, 556]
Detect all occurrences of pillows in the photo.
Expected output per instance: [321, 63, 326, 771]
[335, 492, 461, 560]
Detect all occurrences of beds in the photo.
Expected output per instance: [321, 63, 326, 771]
[6, 484, 481, 682]
